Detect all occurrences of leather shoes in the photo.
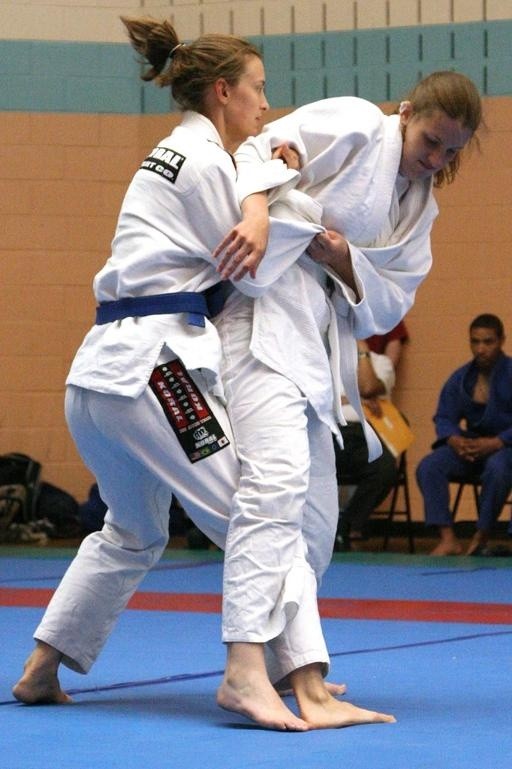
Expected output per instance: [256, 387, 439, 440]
[333, 516, 351, 551]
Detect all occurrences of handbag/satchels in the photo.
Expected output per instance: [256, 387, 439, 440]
[0, 452, 80, 544]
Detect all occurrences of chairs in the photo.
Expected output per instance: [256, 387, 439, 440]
[377, 447, 420, 552]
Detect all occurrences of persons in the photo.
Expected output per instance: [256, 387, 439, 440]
[329, 337, 402, 554]
[362, 318, 410, 371]
[10, 10, 399, 730]
[208, 65, 493, 731]
[415, 312, 512, 556]
[48, 485, 119, 541]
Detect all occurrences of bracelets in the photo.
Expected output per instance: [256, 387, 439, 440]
[358, 351, 372, 361]
